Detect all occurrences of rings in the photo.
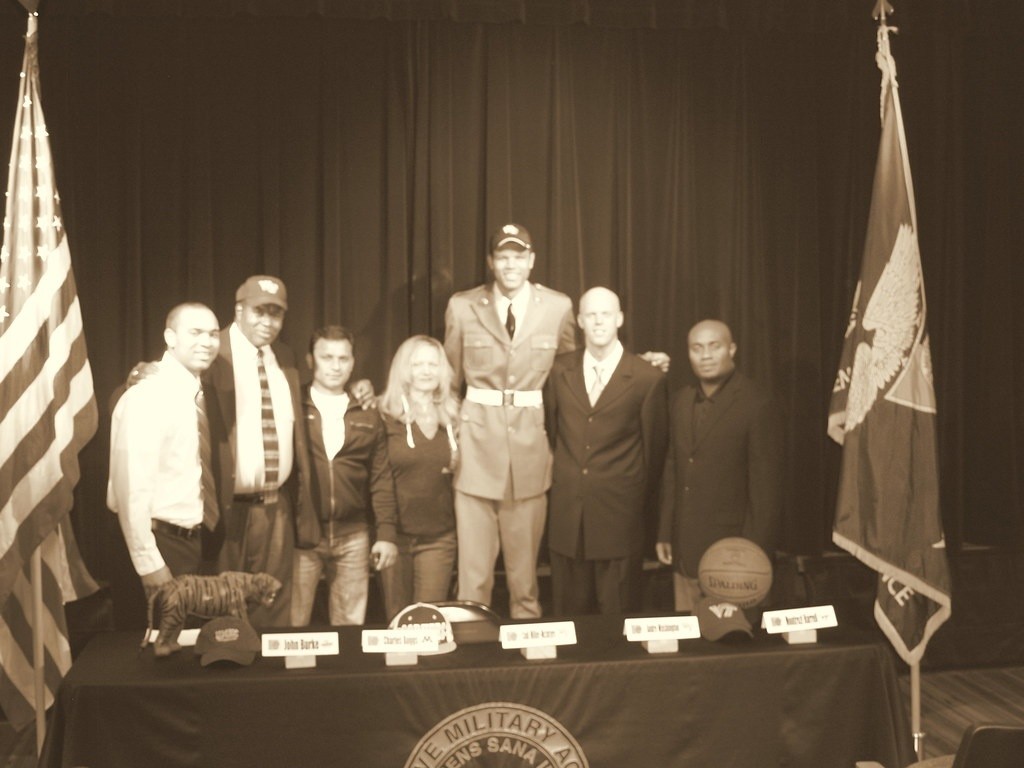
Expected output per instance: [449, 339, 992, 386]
[135, 370, 139, 376]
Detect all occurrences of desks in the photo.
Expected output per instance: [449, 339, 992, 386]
[38, 602, 919, 768]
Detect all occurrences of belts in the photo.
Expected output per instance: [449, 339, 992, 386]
[465, 386, 543, 407]
[149, 519, 203, 539]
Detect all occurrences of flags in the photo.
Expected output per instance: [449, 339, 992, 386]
[829, 59, 953, 664]
[0, 44, 100, 732]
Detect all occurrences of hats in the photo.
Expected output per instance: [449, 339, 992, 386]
[391, 602, 457, 656]
[195, 617, 259, 665]
[489, 223, 532, 255]
[689, 595, 753, 641]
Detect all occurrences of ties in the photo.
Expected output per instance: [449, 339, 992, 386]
[257, 349, 279, 505]
[589, 367, 605, 406]
[506, 302, 517, 340]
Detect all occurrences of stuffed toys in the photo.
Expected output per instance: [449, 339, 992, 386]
[142, 571, 282, 656]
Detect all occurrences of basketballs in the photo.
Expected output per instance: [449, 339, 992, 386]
[699, 536, 774, 610]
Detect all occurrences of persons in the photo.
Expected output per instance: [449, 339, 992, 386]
[291, 327, 399, 629]
[656, 319, 782, 613]
[376, 334, 458, 619]
[543, 287, 668, 613]
[361, 224, 671, 618]
[128, 276, 374, 621]
[106, 303, 221, 631]
